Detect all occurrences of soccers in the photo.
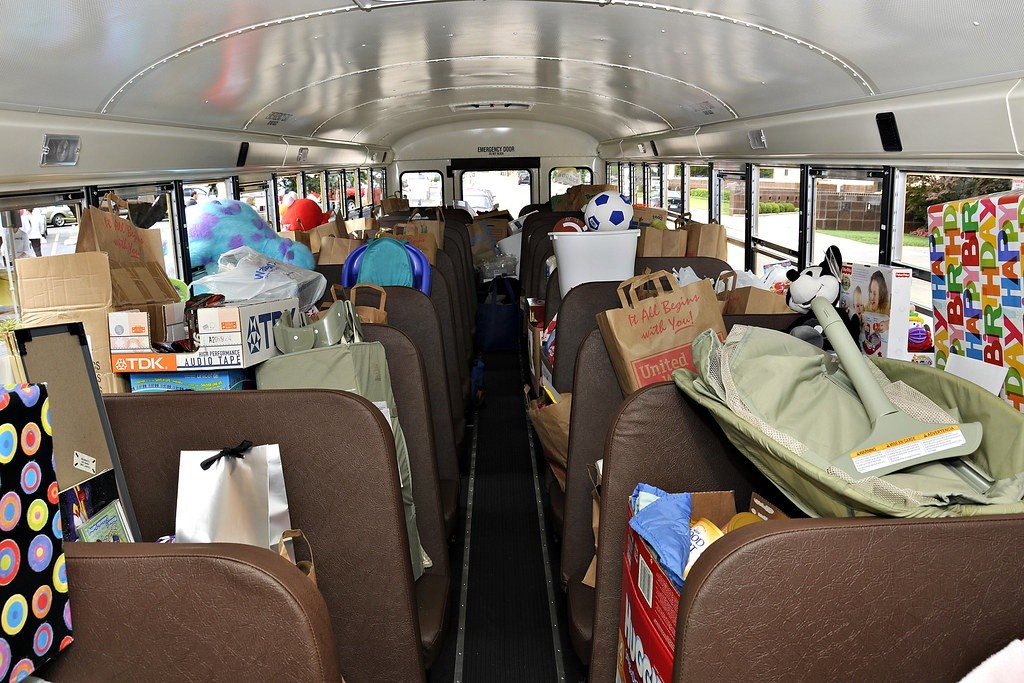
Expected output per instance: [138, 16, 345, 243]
[584, 190, 634, 231]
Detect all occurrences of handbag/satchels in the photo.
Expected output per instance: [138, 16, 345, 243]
[634, 216, 688, 258]
[639, 260, 796, 315]
[317, 234, 363, 265]
[381, 190, 409, 214]
[175, 438, 297, 569]
[551, 170, 618, 211]
[75, 190, 166, 272]
[379, 221, 436, 267]
[581, 464, 602, 590]
[184, 244, 327, 312]
[596, 269, 728, 399]
[473, 276, 520, 352]
[680, 211, 728, 261]
[406, 207, 445, 250]
[461, 208, 496, 270]
[524, 375, 572, 493]
[318, 283, 388, 325]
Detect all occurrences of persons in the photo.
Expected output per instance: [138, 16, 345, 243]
[1, 227, 29, 267]
[187, 191, 198, 206]
[21, 208, 42, 257]
[853, 286, 863, 315]
[246, 196, 257, 212]
[864, 271, 890, 333]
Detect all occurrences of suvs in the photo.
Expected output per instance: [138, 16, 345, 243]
[518, 172, 530, 185]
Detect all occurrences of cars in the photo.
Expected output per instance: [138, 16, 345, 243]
[417, 184, 496, 214]
[650, 195, 682, 213]
[182, 186, 210, 206]
[309, 186, 381, 213]
[31, 203, 77, 227]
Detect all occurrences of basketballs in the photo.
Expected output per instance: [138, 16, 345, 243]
[552, 217, 586, 232]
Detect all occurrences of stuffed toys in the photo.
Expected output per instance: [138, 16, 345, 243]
[785, 245, 861, 351]
[185, 196, 314, 275]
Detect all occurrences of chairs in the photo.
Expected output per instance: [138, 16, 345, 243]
[518, 188, 1024, 683]
[5, 201, 480, 683]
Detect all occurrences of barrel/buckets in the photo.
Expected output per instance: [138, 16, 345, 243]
[547, 229, 642, 299]
[682, 511, 767, 583]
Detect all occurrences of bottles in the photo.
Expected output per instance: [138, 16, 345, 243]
[649, 218, 669, 231]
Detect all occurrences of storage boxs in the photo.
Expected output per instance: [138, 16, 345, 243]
[839, 261, 912, 358]
[14, 250, 182, 394]
[613, 486, 785, 683]
[527, 319, 543, 379]
[473, 210, 513, 247]
[109, 295, 300, 371]
[132, 370, 249, 394]
[544, 228, 641, 294]
